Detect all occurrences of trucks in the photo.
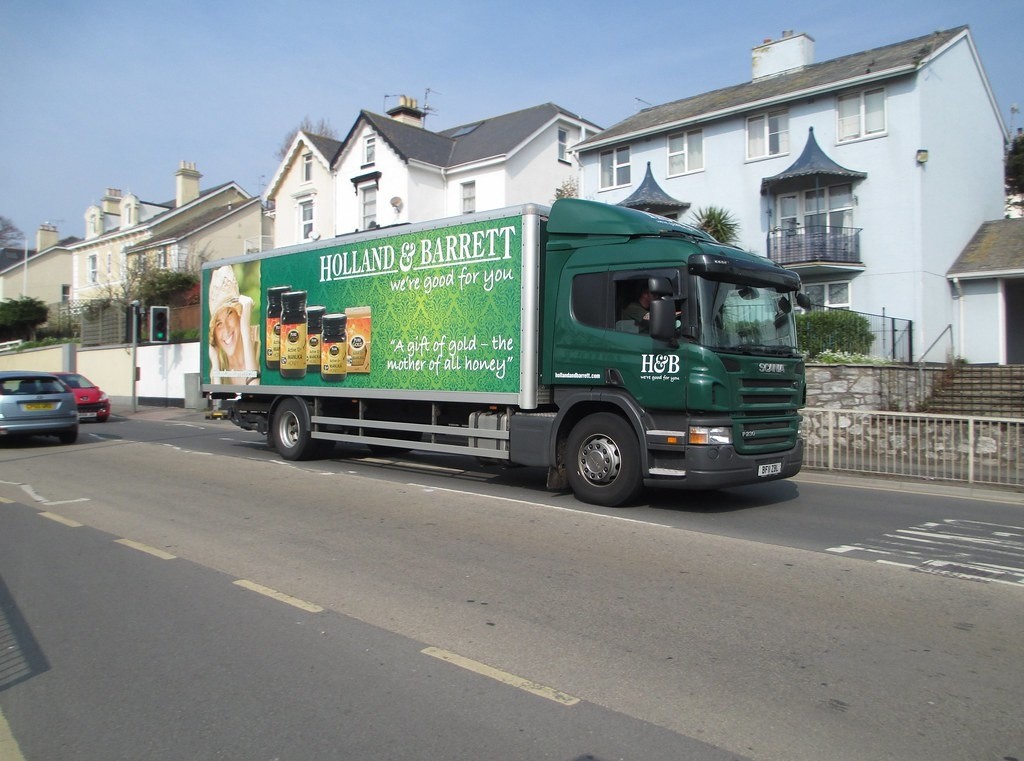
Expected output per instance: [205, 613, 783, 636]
[198, 198, 815, 507]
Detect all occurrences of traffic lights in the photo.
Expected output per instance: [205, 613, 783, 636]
[150, 306, 169, 343]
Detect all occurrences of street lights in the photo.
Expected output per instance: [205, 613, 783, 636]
[19, 235, 29, 300]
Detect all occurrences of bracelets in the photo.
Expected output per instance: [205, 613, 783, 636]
[246, 370, 260, 385]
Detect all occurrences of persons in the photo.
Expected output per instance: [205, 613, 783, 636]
[622, 280, 681, 333]
[208, 265, 260, 385]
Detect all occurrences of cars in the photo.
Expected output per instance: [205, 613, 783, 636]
[0, 370, 78, 444]
[52, 373, 111, 422]
